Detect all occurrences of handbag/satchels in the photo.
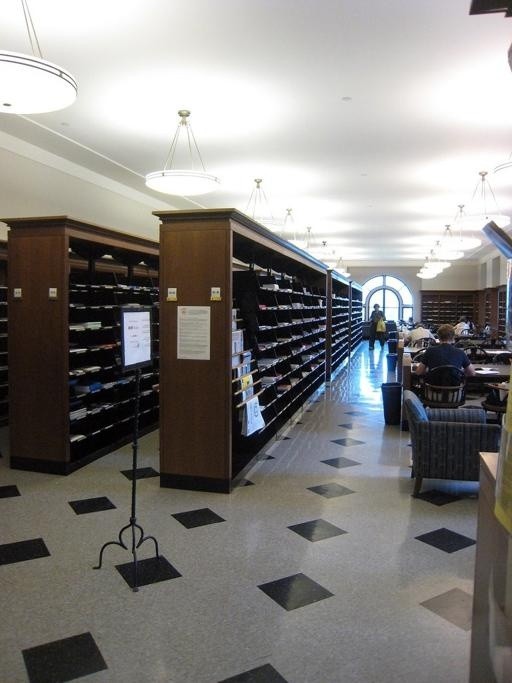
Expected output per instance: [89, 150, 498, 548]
[375, 320, 387, 332]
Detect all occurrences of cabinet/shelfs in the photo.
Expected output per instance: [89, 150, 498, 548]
[0, 207, 362, 496]
[419, 284, 507, 341]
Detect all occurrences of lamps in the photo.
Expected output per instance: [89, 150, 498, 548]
[143, 107, 220, 197]
[240, 178, 276, 232]
[0, 0, 78, 115]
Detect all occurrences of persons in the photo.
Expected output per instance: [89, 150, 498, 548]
[365, 304, 492, 409]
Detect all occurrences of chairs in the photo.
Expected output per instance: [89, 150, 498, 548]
[409, 336, 512, 424]
[401, 388, 501, 499]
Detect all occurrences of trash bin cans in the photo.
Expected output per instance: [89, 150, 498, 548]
[387, 353, 398, 372]
[387, 331, 398, 354]
[382, 382, 401, 425]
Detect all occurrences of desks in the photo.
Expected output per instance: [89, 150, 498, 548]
[397, 346, 511, 431]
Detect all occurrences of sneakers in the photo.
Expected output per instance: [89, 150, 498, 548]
[369, 342, 385, 350]
